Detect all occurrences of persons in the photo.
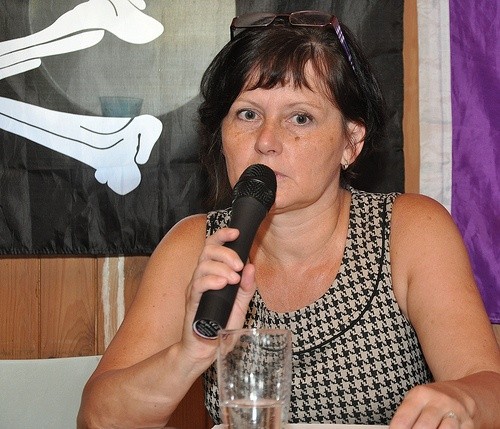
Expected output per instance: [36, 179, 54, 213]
[76, 12, 499, 428]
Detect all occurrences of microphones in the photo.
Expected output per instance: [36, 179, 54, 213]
[192, 164, 277, 341]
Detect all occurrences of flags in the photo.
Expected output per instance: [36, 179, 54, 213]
[414, 0, 500, 326]
[0, 0, 404, 259]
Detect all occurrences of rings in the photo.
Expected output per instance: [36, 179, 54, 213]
[446, 413, 459, 421]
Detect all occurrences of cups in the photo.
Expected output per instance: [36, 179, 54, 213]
[217, 328, 292, 429]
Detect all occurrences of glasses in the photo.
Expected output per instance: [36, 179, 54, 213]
[229, 10, 358, 79]
